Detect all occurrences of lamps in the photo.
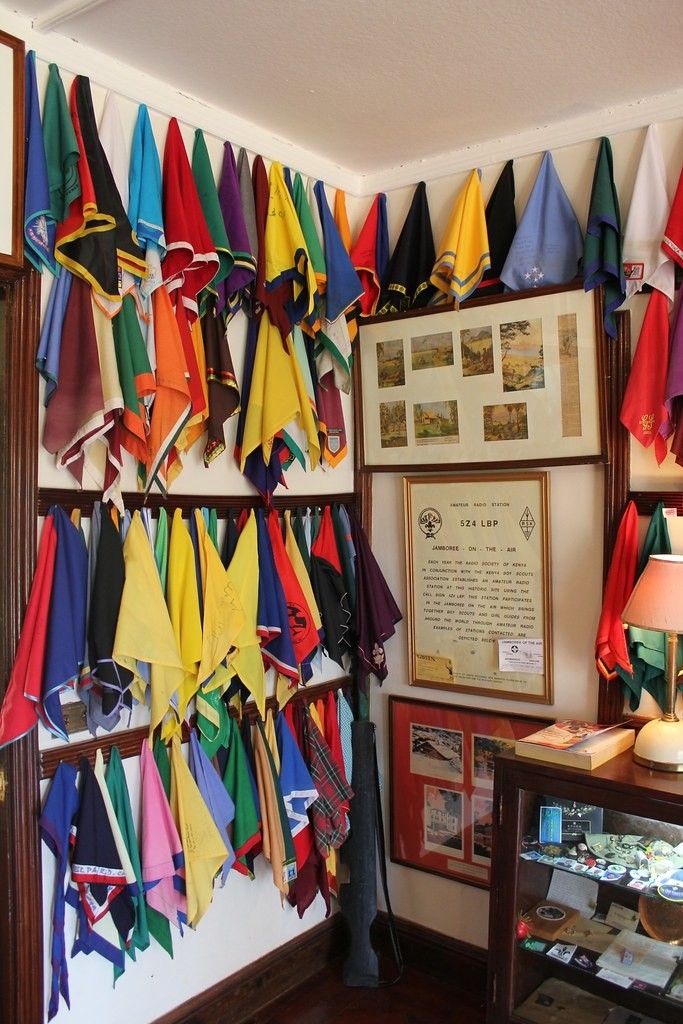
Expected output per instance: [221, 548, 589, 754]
[620, 555, 683, 774]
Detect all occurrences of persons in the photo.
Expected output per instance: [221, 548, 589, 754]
[518, 848, 649, 895]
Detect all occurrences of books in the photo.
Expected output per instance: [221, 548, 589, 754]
[514, 718, 636, 771]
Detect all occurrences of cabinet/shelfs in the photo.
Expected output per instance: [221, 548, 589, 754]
[485, 748, 683, 1023]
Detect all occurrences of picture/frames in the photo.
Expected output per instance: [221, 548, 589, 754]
[388, 695, 555, 894]
[355, 276, 612, 475]
